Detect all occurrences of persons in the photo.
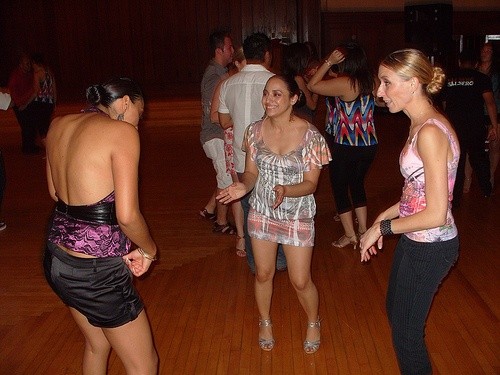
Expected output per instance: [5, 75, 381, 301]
[283, 42, 319, 123]
[463, 43, 500, 193]
[218, 33, 287, 274]
[197, 29, 239, 235]
[209, 48, 248, 258]
[307, 41, 380, 249]
[358, 49, 459, 375]
[216, 74, 334, 354]
[433, 50, 496, 212]
[9, 56, 42, 153]
[305, 42, 317, 66]
[31, 51, 57, 142]
[44, 77, 157, 375]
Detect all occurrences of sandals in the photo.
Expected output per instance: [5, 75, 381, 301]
[212, 221, 238, 235]
[200, 208, 217, 222]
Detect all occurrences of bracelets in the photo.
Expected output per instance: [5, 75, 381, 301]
[325, 60, 332, 67]
[138, 248, 154, 261]
[380, 219, 393, 235]
[140, 248, 157, 260]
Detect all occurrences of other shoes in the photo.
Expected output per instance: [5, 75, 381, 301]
[21, 146, 45, 154]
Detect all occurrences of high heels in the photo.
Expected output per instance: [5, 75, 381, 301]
[304, 314, 322, 354]
[235, 235, 247, 258]
[331, 234, 357, 250]
[258, 315, 275, 352]
